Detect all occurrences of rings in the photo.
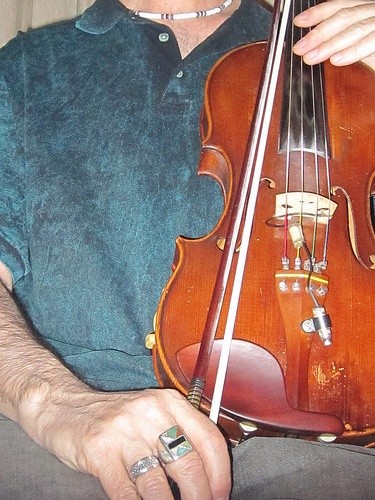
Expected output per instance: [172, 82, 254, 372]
[158, 426, 194, 462]
[129, 456, 159, 478]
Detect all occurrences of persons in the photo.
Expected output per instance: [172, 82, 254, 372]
[0, 0, 375, 500]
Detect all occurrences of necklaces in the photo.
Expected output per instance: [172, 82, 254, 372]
[134, 0, 232, 19]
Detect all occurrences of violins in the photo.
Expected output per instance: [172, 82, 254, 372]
[146, 1, 375, 448]
